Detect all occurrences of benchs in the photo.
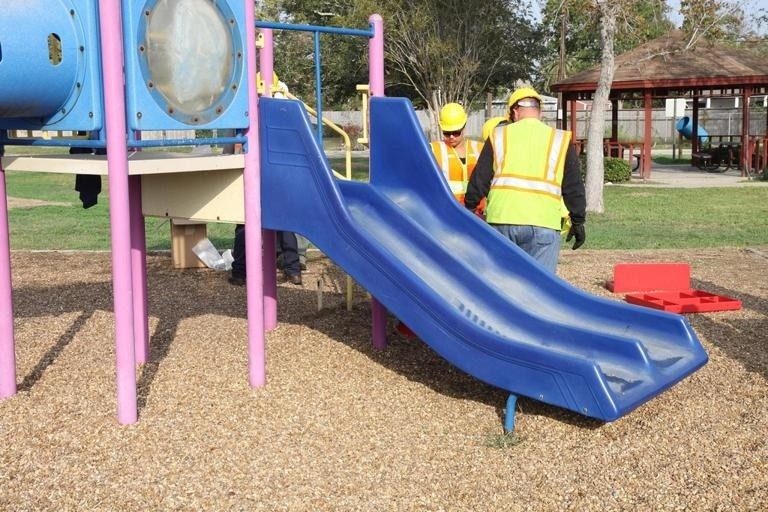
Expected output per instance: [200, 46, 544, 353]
[632, 154, 641, 171]
[690, 152, 756, 172]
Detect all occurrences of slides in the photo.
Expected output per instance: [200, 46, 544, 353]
[259, 97, 708, 422]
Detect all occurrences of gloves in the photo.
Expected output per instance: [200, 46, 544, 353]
[565, 220, 586, 250]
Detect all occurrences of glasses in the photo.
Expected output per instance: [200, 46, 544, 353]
[442, 130, 462, 138]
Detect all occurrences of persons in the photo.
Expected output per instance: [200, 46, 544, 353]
[227, 68, 303, 286]
[274, 83, 308, 270]
[464, 88, 587, 274]
[428, 100, 490, 217]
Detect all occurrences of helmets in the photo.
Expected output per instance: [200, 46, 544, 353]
[507, 87, 545, 116]
[256, 69, 280, 95]
[438, 102, 469, 132]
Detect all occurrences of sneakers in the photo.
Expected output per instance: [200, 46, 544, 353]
[226, 259, 308, 286]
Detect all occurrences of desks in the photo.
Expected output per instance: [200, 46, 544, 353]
[603, 144, 624, 159]
[709, 141, 743, 172]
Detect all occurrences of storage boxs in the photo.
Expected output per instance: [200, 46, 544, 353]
[170, 219, 207, 268]
